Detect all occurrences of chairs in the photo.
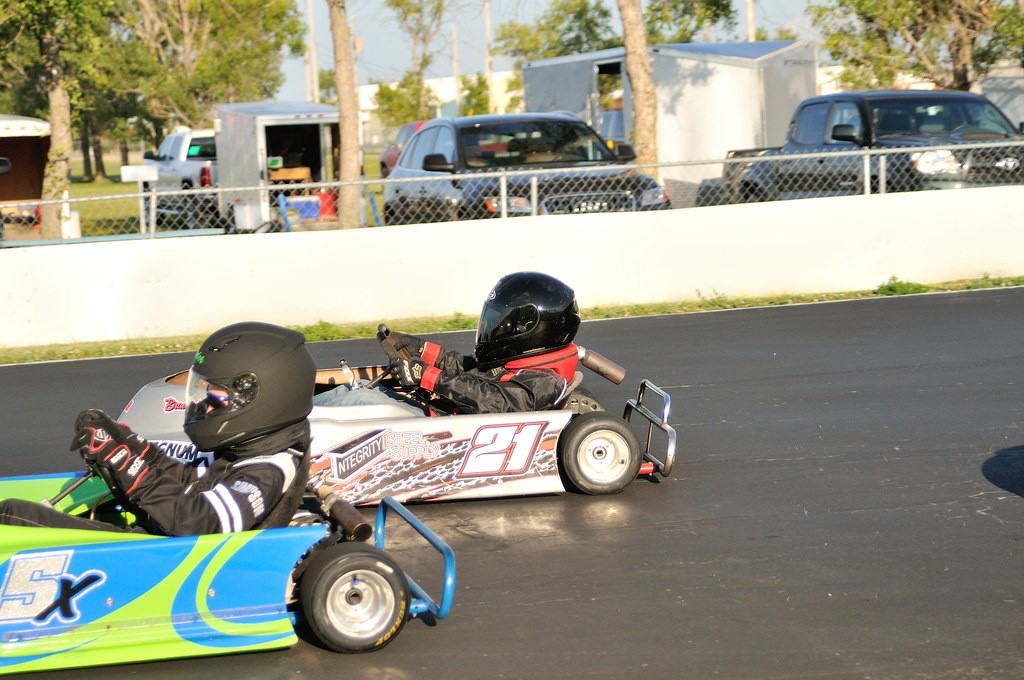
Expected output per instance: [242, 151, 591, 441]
[882, 104, 984, 137]
[466, 138, 558, 166]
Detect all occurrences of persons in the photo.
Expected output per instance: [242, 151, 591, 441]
[312, 271, 581, 417]
[0, 322, 318, 537]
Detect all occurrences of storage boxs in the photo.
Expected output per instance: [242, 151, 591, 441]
[285, 197, 320, 218]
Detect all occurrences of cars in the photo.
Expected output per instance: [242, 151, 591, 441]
[383, 110, 671, 226]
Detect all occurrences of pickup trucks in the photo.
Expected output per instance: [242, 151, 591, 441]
[140, 126, 218, 230]
[723, 88, 1024, 204]
[379, 120, 513, 177]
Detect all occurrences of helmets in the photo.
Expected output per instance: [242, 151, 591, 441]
[183, 323, 317, 451]
[476, 272, 581, 372]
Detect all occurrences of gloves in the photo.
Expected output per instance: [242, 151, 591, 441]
[394, 332, 428, 357]
[389, 356, 428, 387]
[70, 409, 160, 497]
